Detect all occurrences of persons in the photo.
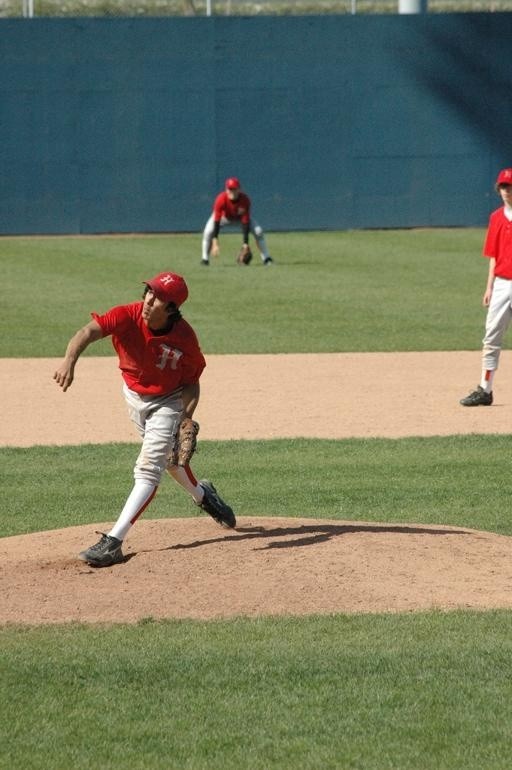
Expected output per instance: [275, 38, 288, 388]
[200, 177, 273, 265]
[459, 167, 512, 407]
[52, 272, 236, 568]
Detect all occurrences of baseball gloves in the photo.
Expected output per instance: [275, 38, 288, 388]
[171, 419, 199, 468]
[238, 247, 251, 265]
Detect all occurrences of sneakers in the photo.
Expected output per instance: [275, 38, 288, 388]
[199, 257, 210, 267]
[458, 388, 496, 408]
[76, 535, 126, 568]
[258, 255, 274, 266]
[192, 478, 237, 533]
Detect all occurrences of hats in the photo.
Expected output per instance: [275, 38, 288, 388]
[225, 176, 238, 190]
[143, 268, 189, 308]
[495, 166, 512, 191]
